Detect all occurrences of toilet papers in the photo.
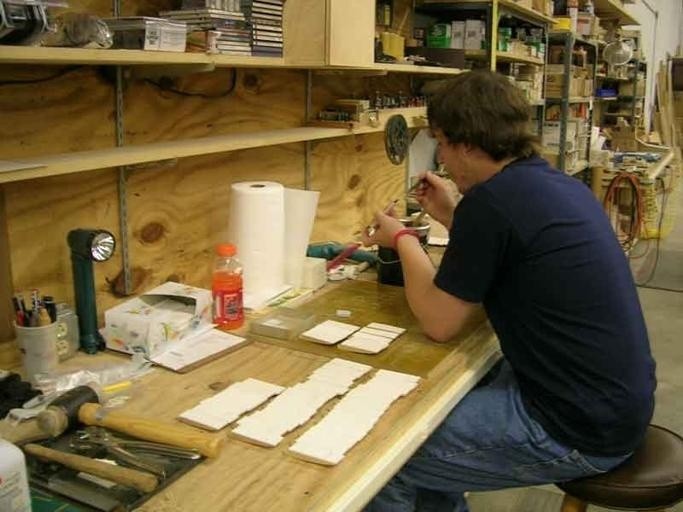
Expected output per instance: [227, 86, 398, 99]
[230, 181, 320, 307]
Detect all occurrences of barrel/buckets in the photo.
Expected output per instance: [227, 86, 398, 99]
[376, 221, 430, 285]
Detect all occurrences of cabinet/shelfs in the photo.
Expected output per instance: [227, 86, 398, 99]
[482, 1, 650, 181]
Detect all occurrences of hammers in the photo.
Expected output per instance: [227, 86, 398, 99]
[37, 381, 226, 461]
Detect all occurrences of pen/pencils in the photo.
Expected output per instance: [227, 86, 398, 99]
[11, 287, 56, 328]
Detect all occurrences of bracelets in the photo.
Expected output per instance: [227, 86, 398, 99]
[392, 229, 420, 254]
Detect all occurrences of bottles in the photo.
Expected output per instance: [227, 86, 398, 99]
[577, 46, 585, 68]
[210, 244, 246, 331]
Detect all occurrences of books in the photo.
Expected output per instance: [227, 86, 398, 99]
[100, 0, 289, 61]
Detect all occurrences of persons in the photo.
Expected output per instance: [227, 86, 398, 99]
[359, 66, 660, 512]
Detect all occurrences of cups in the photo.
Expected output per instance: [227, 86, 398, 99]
[12, 318, 59, 378]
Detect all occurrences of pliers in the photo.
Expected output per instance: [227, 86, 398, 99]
[68, 426, 200, 481]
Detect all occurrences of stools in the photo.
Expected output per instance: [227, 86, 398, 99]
[549, 422, 682, 512]
